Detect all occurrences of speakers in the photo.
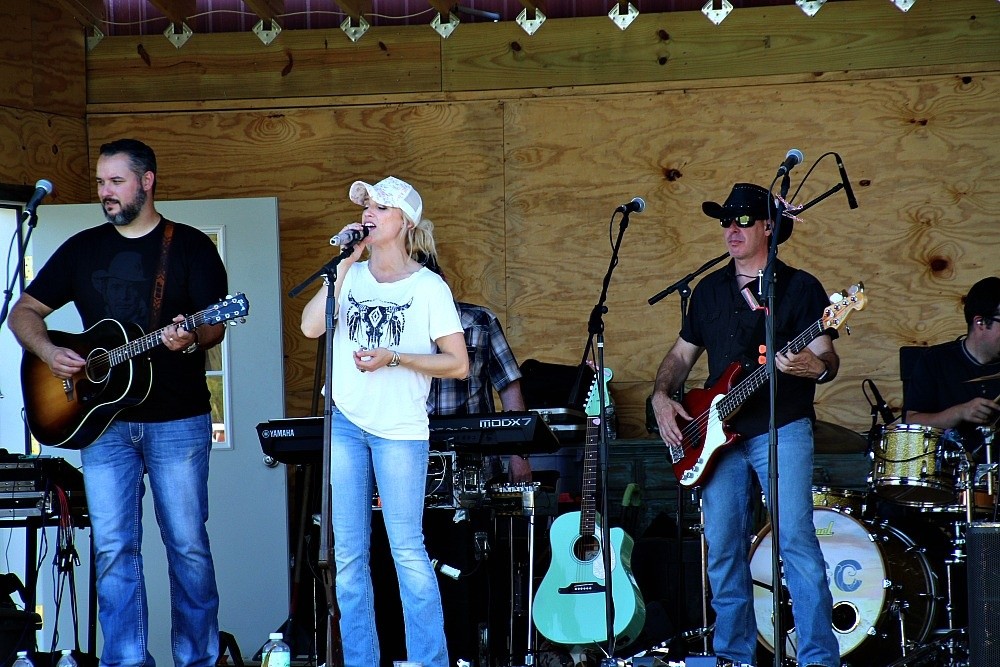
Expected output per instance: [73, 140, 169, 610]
[966, 521, 1000, 667]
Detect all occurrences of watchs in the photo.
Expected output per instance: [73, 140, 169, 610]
[811, 359, 829, 384]
[386, 351, 400, 367]
[182, 331, 200, 354]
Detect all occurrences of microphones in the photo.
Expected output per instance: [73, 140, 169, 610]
[330, 224, 370, 246]
[616, 197, 646, 213]
[777, 149, 803, 177]
[835, 153, 858, 209]
[21, 179, 53, 222]
[868, 379, 896, 424]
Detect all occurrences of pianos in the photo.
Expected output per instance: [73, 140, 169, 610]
[253, 409, 562, 667]
[0, 448, 98, 667]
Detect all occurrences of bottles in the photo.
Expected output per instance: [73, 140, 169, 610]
[56, 649, 77, 667]
[261, 632, 290, 667]
[12, 651, 34, 667]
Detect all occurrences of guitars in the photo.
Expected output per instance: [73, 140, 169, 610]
[667, 281, 870, 490]
[531, 367, 648, 655]
[20, 290, 250, 452]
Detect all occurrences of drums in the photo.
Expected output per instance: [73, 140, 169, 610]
[871, 422, 997, 513]
[744, 485, 939, 667]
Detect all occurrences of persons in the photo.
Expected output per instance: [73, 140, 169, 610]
[901, 277, 1000, 464]
[412, 249, 532, 487]
[651, 183, 840, 667]
[301, 176, 469, 667]
[6, 140, 228, 667]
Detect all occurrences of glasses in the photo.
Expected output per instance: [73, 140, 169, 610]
[719, 212, 769, 228]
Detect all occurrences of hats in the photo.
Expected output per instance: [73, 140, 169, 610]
[349, 176, 422, 229]
[702, 183, 794, 246]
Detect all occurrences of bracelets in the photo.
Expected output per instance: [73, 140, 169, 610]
[518, 454, 530, 460]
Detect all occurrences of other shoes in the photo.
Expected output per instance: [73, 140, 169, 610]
[716, 657, 740, 667]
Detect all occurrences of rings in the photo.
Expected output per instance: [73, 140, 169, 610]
[360, 369, 366, 373]
[789, 366, 793, 371]
[169, 337, 173, 341]
